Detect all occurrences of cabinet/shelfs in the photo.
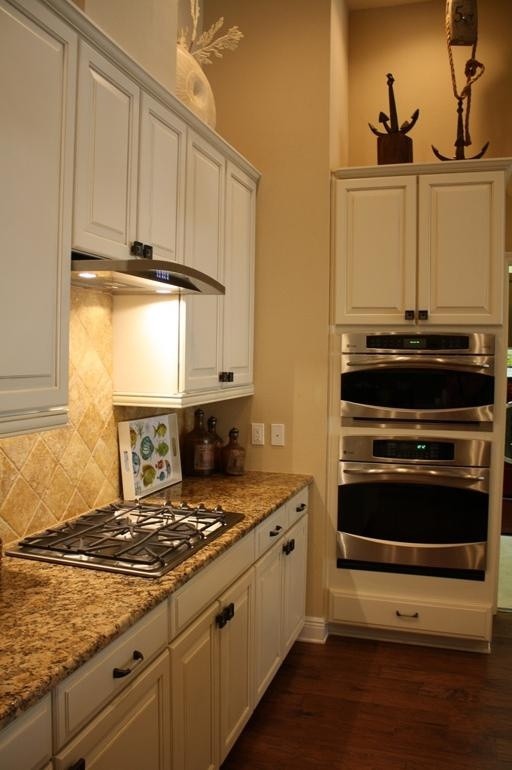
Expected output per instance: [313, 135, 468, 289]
[71, 19, 190, 265]
[112, 115, 261, 413]
[253, 483, 310, 719]
[0, 685, 53, 770]
[2, 2, 86, 442]
[52, 596, 167, 770]
[329, 156, 511, 328]
[167, 529, 252, 770]
[325, 567, 496, 657]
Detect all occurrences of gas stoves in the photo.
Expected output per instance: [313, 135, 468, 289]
[6, 496, 250, 586]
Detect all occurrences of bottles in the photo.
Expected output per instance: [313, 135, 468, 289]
[205, 414, 225, 475]
[221, 427, 248, 477]
[183, 407, 216, 479]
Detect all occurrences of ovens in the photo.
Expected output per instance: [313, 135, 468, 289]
[334, 329, 498, 586]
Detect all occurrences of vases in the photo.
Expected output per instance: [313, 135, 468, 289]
[171, 36, 225, 131]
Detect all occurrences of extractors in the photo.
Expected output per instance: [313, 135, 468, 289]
[70, 256, 228, 299]
